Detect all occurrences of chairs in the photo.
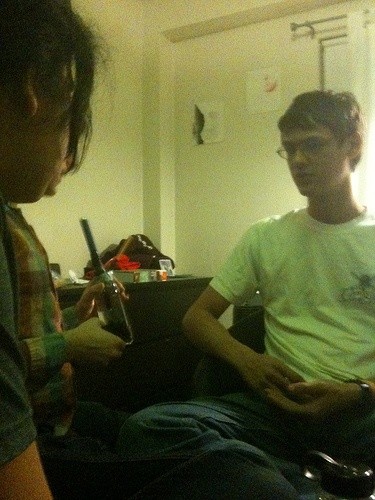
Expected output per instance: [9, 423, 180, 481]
[210, 307, 265, 398]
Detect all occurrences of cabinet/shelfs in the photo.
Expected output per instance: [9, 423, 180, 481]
[57, 275, 214, 414]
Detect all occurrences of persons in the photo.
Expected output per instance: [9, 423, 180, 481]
[120, 91, 375, 500]
[0, 103, 133, 480]
[0, 0, 96, 500]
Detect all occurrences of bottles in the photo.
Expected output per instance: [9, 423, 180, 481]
[80, 220, 133, 344]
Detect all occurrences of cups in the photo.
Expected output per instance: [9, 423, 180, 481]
[300, 450, 375, 500]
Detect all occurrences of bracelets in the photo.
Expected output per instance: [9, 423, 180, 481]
[344, 379, 370, 403]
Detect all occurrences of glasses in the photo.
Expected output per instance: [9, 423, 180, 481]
[276, 142, 337, 160]
